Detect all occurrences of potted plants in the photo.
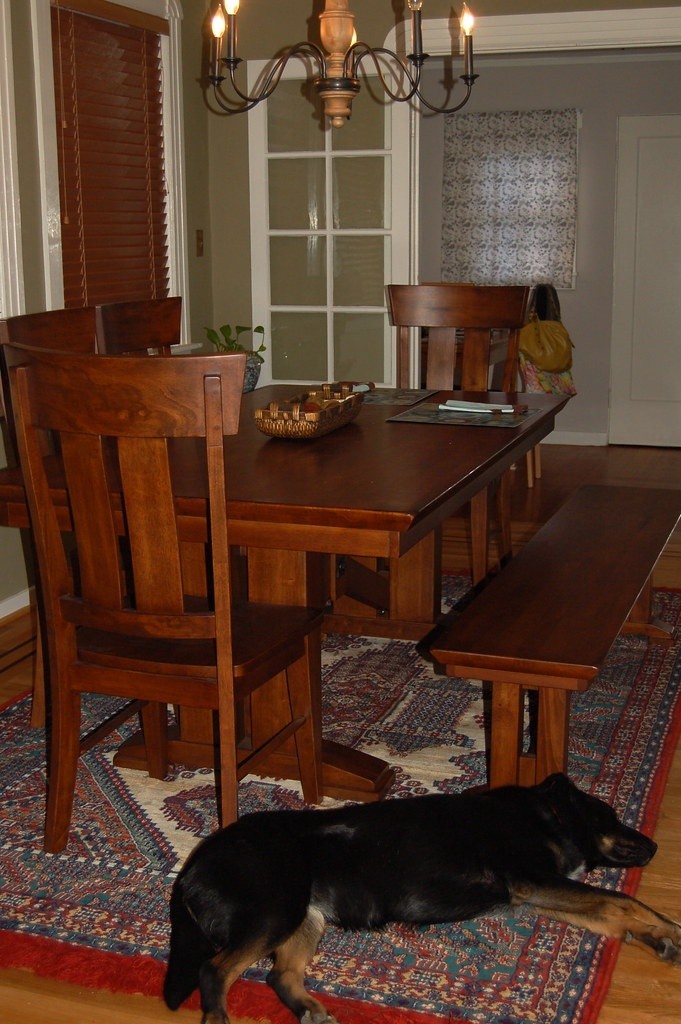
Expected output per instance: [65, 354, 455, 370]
[203, 323, 267, 392]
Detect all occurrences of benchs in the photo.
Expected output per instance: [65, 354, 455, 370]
[429, 482, 681, 788]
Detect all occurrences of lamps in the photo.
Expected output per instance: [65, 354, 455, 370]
[208, 0, 480, 128]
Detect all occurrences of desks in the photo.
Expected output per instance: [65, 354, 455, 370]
[0, 385, 571, 804]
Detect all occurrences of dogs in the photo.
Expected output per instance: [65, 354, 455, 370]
[164, 770, 681, 1024]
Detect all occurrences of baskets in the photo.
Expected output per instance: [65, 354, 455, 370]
[255, 385, 365, 440]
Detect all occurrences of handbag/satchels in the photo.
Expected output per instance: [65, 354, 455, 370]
[517, 282, 577, 399]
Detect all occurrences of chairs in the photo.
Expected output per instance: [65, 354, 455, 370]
[4, 341, 328, 853]
[0, 295, 185, 732]
[387, 285, 532, 587]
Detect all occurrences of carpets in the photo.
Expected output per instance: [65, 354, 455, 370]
[0, 569, 681, 1024]
